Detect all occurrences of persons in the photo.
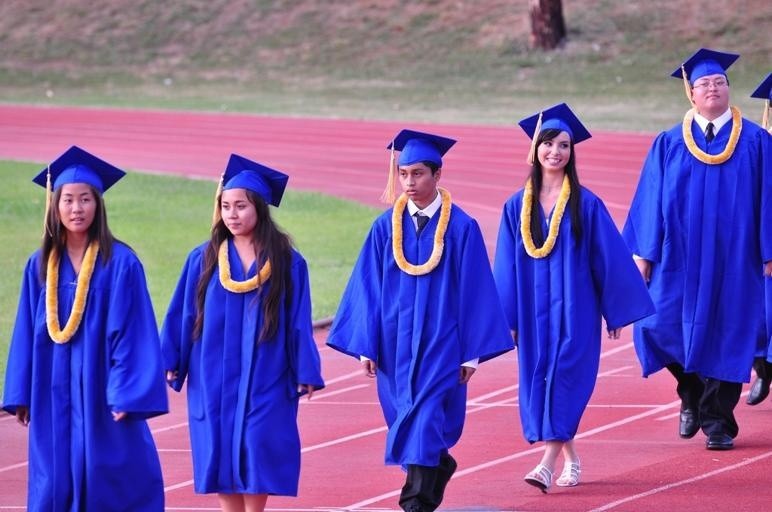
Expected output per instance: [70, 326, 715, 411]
[160, 153, 326, 512]
[620, 47, 772, 452]
[326, 128, 516, 512]
[746, 74, 772, 405]
[1, 143, 170, 512]
[492, 103, 658, 495]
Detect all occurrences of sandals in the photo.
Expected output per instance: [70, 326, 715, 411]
[555, 458, 582, 487]
[523, 464, 554, 494]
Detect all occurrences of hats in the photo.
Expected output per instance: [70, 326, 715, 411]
[750, 69, 772, 124]
[518, 102, 592, 167]
[672, 48, 741, 109]
[210, 153, 288, 232]
[32, 144, 126, 240]
[380, 129, 458, 203]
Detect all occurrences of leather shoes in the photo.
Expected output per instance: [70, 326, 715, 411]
[746, 376, 771, 406]
[707, 432, 734, 450]
[679, 408, 701, 438]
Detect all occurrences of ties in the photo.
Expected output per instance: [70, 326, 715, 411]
[705, 123, 714, 144]
[414, 212, 430, 237]
